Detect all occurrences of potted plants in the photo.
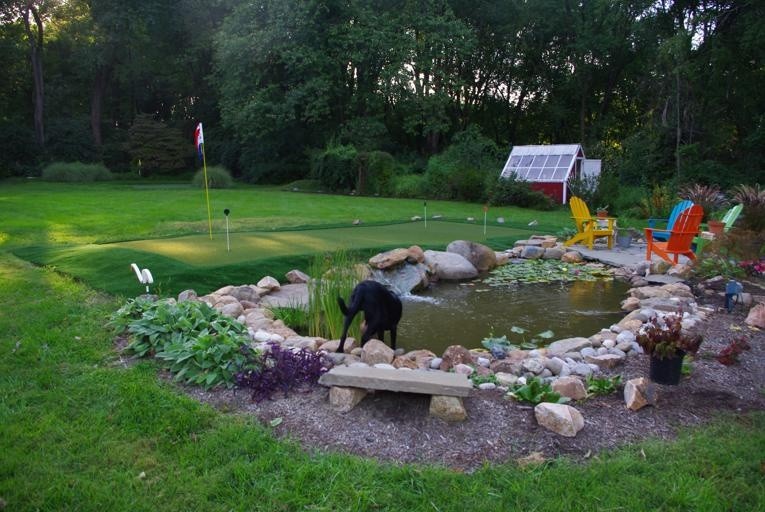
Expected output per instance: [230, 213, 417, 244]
[636, 302, 706, 388]
[617, 215, 636, 248]
[706, 209, 726, 236]
[594, 203, 608, 219]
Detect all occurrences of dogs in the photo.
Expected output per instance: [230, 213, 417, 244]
[335, 279, 403, 354]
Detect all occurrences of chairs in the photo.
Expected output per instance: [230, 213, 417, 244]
[645, 204, 706, 266]
[564, 196, 614, 251]
[648, 201, 696, 252]
[693, 204, 744, 262]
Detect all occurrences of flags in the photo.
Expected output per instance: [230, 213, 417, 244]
[192, 122, 204, 162]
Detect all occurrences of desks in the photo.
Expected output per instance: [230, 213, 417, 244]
[595, 218, 618, 238]
[700, 232, 716, 243]
